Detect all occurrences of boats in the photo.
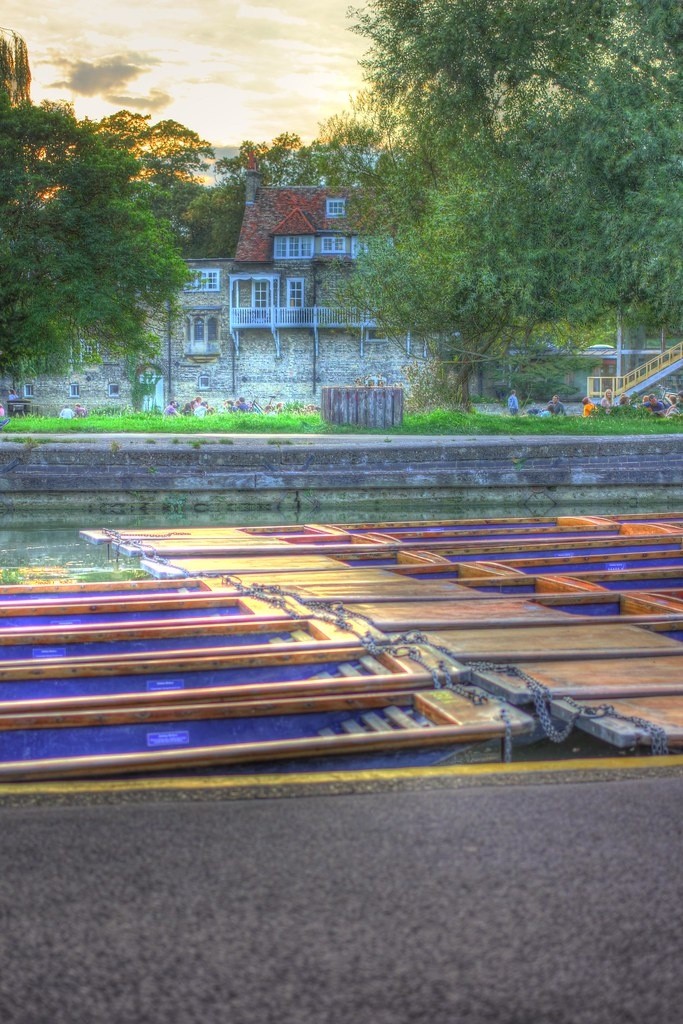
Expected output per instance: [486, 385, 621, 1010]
[1, 499, 682, 781]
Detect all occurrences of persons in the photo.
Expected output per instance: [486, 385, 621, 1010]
[163, 400, 181, 416]
[60, 404, 75, 419]
[602, 389, 613, 414]
[508, 390, 518, 416]
[264, 402, 287, 417]
[8, 389, 19, 400]
[527, 396, 567, 417]
[74, 403, 88, 418]
[0, 404, 4, 416]
[221, 397, 253, 413]
[582, 397, 598, 417]
[614, 393, 637, 409]
[638, 391, 683, 418]
[300, 404, 321, 415]
[181, 397, 214, 418]
